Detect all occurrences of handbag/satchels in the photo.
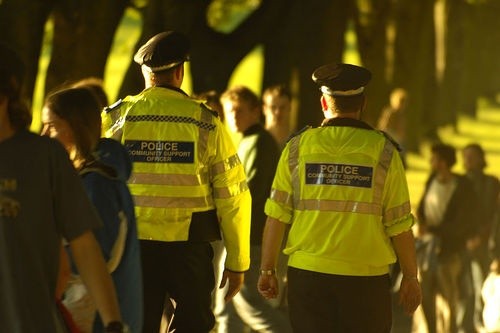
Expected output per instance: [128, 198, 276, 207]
[61, 271, 97, 333]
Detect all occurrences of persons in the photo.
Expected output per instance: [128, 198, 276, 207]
[441, 144, 499, 332]
[377, 88, 409, 165]
[39, 87, 144, 333]
[216, 85, 292, 332]
[100, 30, 253, 332]
[0, 61, 127, 333]
[258, 63, 422, 333]
[416, 143, 462, 331]
[259, 88, 292, 307]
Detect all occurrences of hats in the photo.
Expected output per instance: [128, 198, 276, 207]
[312, 64, 371, 96]
[132, 31, 193, 74]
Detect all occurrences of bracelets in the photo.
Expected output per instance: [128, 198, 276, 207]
[260, 269, 275, 276]
[106, 321, 124, 333]
